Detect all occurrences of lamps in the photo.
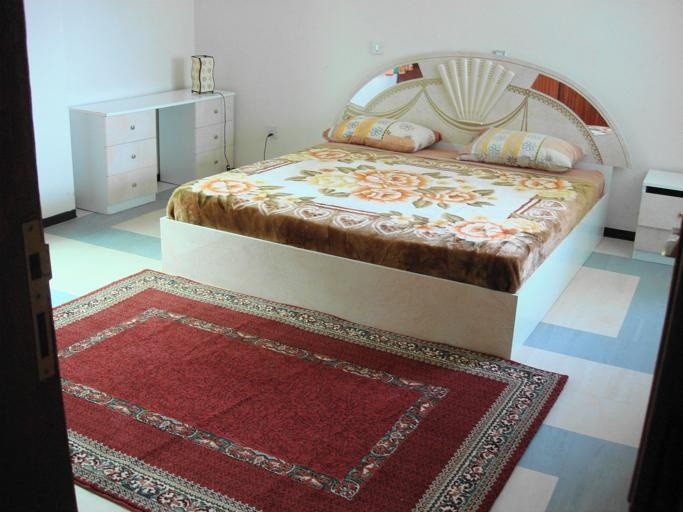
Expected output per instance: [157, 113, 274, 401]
[187, 54, 215, 96]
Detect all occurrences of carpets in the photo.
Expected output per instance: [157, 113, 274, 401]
[49, 266, 568, 512]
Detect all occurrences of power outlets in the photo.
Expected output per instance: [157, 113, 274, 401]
[267, 126, 280, 140]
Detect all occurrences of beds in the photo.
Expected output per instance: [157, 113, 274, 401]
[160, 52, 632, 361]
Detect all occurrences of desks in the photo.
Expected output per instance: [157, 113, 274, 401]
[69, 88, 234, 216]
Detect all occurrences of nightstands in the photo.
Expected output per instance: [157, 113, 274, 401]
[635, 170, 683, 259]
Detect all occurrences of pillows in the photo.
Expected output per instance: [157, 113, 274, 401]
[323, 114, 443, 155]
[455, 125, 587, 175]
